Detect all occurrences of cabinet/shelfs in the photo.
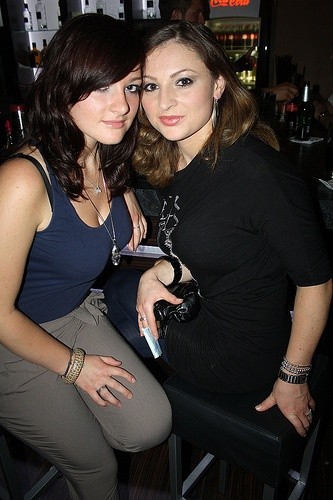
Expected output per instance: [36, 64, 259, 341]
[0, 0, 161, 81]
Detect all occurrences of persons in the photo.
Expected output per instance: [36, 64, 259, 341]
[139, 0, 298, 111]
[0, 13, 172, 500]
[102, 20, 333, 438]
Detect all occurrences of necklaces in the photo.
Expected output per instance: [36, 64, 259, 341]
[83, 171, 102, 194]
[80, 166, 122, 266]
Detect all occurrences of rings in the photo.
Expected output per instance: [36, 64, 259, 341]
[139, 316, 147, 321]
[305, 409, 313, 417]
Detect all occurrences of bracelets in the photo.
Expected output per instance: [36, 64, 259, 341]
[278, 369, 309, 384]
[282, 357, 312, 374]
[62, 348, 85, 384]
[154, 256, 182, 287]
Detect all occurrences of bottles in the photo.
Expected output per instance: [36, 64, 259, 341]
[22, 4, 32, 30]
[35, 0, 47, 29]
[96, 0, 103, 14]
[285, 98, 300, 136]
[295, 81, 313, 141]
[147, 0, 156, 19]
[209, 22, 258, 48]
[31, 43, 40, 64]
[58, 0, 61, 29]
[27, 47, 36, 67]
[40, 39, 48, 60]
[84, 0, 92, 13]
[118, 0, 124, 21]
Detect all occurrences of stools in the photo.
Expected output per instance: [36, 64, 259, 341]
[161, 369, 330, 500]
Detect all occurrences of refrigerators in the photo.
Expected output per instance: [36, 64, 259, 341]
[203, 0, 273, 90]
